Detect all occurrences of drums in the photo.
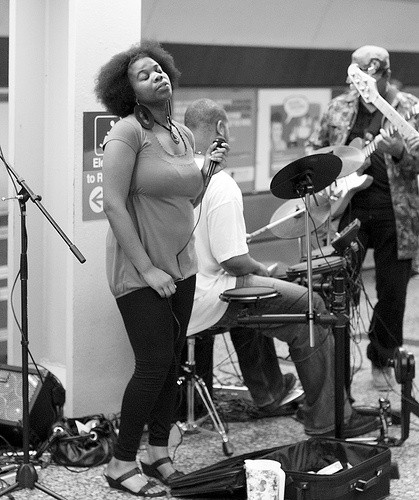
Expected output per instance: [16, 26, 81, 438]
[219, 287, 279, 300]
[285, 255, 347, 277]
[302, 245, 336, 261]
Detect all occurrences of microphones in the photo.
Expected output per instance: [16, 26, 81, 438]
[205, 135, 227, 187]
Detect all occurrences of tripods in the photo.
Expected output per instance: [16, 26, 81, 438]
[0, 152, 87, 499]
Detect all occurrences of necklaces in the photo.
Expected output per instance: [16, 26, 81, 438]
[153, 117, 180, 144]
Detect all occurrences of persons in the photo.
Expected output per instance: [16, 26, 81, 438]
[306, 45, 419, 391]
[183, 98, 383, 439]
[93, 40, 230, 497]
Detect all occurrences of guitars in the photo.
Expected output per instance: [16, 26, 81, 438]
[346, 63, 419, 139]
[318, 102, 419, 216]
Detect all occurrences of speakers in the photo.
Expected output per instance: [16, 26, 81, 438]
[0, 363, 66, 447]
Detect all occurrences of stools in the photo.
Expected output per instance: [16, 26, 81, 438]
[176, 327, 232, 456]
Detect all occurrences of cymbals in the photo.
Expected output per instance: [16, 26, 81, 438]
[311, 145, 364, 179]
[270, 152, 343, 199]
[269, 194, 331, 239]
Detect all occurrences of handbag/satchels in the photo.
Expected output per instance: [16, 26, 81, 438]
[48, 414, 118, 473]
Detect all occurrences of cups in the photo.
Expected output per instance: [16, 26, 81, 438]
[244, 460, 285, 500]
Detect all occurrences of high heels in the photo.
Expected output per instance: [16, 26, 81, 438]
[140, 457, 185, 486]
[103, 466, 167, 497]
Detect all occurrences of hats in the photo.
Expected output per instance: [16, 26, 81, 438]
[345, 45, 390, 83]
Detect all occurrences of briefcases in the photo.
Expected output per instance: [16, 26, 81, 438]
[171, 436, 395, 500]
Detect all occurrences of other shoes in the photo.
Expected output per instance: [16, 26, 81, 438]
[259, 373, 296, 413]
[311, 411, 380, 438]
[372, 366, 395, 391]
